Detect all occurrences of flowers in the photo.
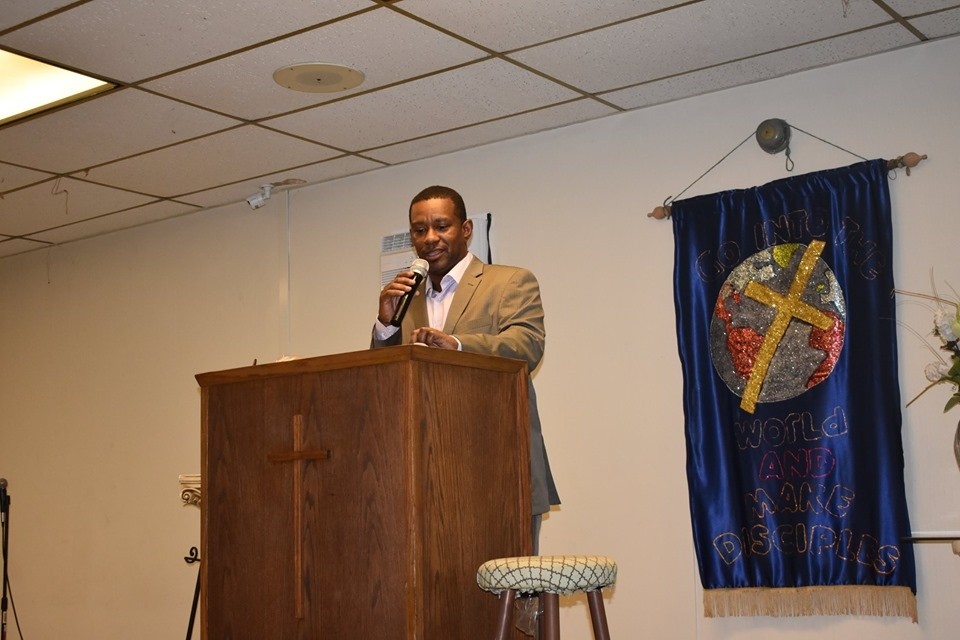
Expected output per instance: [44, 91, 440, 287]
[876, 266, 960, 414]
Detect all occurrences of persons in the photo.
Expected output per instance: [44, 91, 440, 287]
[369, 185, 563, 557]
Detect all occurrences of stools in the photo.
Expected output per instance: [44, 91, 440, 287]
[476, 553, 618, 640]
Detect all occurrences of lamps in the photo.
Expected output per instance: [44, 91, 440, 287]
[244, 183, 273, 210]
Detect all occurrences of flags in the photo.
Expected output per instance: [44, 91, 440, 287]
[671, 159, 919, 626]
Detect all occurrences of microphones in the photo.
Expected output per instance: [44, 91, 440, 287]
[390, 259, 430, 327]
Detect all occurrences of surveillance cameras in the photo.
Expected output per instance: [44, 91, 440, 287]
[245, 192, 266, 210]
